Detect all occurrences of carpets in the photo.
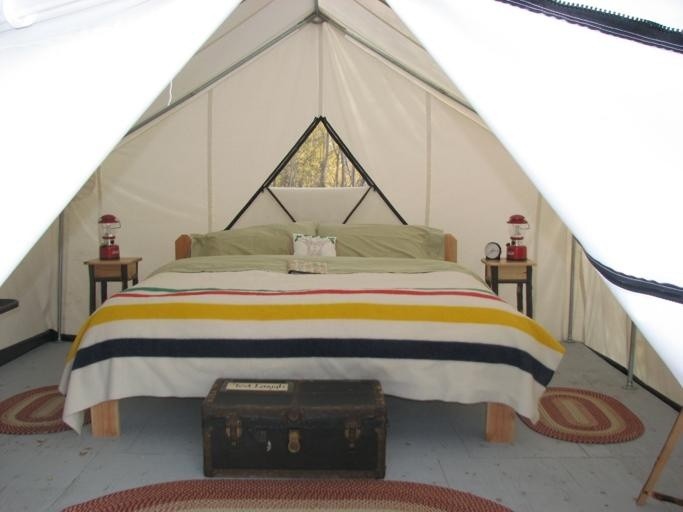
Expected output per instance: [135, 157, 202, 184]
[59, 474, 513, 512]
[0, 383, 89, 435]
[515, 387, 645, 445]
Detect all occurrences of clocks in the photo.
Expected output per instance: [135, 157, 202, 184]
[485, 242, 501, 259]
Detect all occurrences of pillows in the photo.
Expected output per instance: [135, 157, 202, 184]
[189, 222, 446, 257]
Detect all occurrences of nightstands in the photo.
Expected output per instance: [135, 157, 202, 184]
[481, 257, 537, 319]
[83, 256, 143, 317]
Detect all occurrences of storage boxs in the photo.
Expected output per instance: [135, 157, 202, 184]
[199, 376, 388, 478]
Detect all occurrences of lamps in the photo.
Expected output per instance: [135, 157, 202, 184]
[505, 214, 531, 262]
[98, 213, 122, 261]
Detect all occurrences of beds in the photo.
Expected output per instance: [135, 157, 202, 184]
[59, 221, 566, 441]
